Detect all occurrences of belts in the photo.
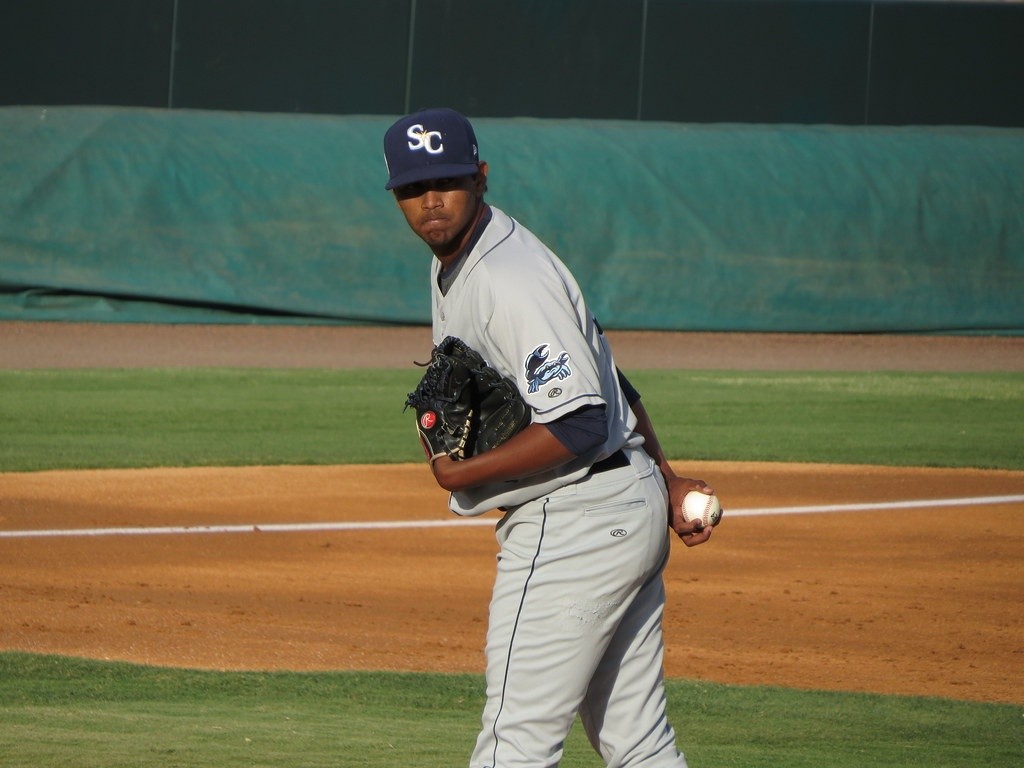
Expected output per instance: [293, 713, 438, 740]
[496, 449, 631, 512]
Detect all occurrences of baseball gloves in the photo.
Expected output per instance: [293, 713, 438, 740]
[408, 333, 533, 477]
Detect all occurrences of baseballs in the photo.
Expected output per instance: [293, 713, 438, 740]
[680, 490, 722, 532]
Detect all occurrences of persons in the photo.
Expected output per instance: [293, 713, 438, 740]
[383, 110, 722, 768]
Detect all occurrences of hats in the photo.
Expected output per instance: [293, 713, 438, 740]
[384, 108, 479, 190]
